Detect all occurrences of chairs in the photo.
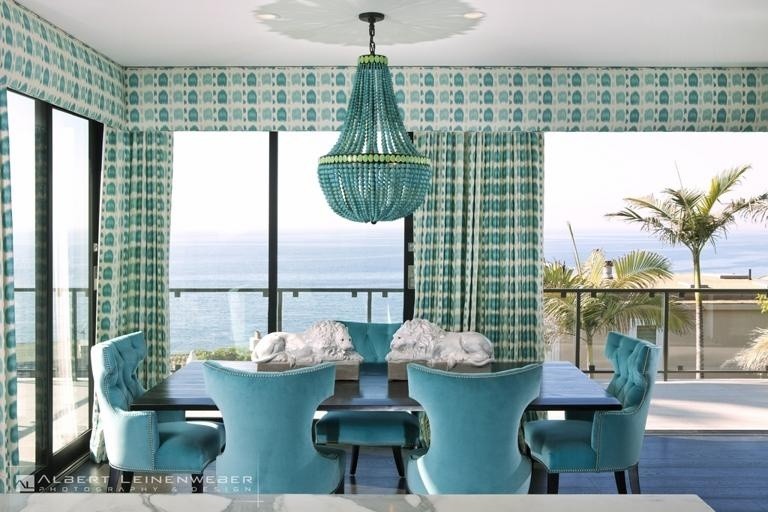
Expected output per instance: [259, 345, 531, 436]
[90, 317, 659, 498]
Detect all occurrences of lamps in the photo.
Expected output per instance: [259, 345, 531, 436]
[316, 10, 431, 224]
